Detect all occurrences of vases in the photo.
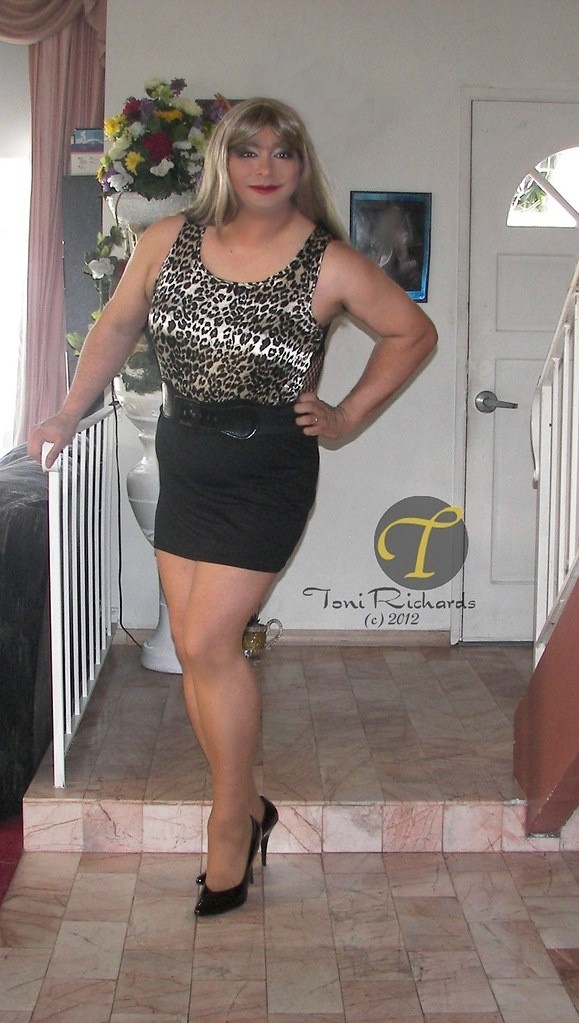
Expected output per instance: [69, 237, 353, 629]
[104, 193, 189, 233]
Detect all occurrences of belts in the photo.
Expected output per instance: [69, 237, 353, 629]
[158, 377, 298, 441]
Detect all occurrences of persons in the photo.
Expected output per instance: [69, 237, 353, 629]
[28, 97, 439, 917]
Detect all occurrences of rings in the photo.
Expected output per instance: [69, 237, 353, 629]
[314, 417, 318, 422]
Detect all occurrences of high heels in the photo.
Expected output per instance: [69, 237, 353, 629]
[193, 814, 260, 916]
[196, 795, 280, 863]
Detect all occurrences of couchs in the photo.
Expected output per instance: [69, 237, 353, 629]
[1, 432, 93, 899]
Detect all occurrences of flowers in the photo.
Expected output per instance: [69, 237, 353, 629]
[65, 78, 231, 392]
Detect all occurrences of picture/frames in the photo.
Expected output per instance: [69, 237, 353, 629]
[350, 189, 432, 301]
